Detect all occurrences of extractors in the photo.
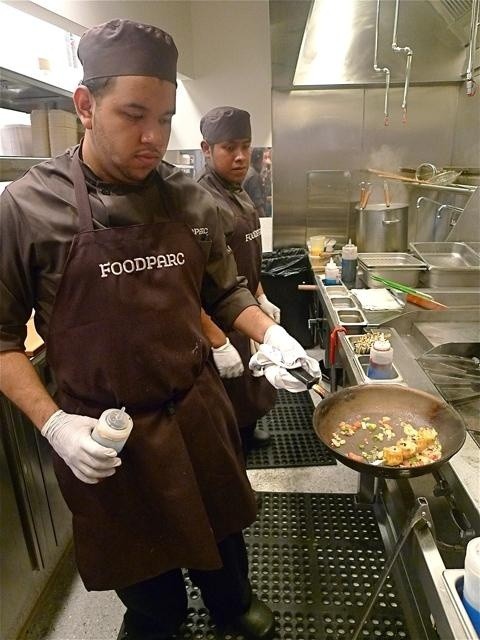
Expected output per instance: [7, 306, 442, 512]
[268, 0, 479, 91]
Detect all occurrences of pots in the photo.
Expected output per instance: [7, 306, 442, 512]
[287, 367, 466, 480]
[354, 202, 410, 253]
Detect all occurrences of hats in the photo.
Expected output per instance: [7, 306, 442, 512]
[76, 18, 180, 84]
[198, 104, 252, 147]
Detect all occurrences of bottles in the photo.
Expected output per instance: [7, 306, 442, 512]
[91, 407, 134, 455]
[367, 331, 394, 380]
[325, 257, 341, 286]
[342, 238, 357, 290]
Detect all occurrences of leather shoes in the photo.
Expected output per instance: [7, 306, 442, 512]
[246, 424, 271, 449]
[224, 596, 276, 639]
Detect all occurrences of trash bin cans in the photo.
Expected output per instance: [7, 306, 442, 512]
[257, 247, 315, 350]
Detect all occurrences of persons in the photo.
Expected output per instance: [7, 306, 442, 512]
[243, 146, 271, 220]
[190, 102, 283, 451]
[0, 13, 329, 640]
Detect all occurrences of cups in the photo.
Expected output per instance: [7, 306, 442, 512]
[310, 235, 325, 256]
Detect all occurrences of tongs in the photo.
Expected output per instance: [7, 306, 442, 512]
[350, 497, 438, 639]
[421, 354, 480, 406]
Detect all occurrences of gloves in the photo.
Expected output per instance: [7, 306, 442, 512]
[210, 337, 245, 380]
[262, 326, 305, 367]
[38, 409, 122, 484]
[259, 291, 281, 324]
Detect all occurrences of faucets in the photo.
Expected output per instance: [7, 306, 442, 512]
[416, 197, 463, 213]
[436, 205, 464, 218]
